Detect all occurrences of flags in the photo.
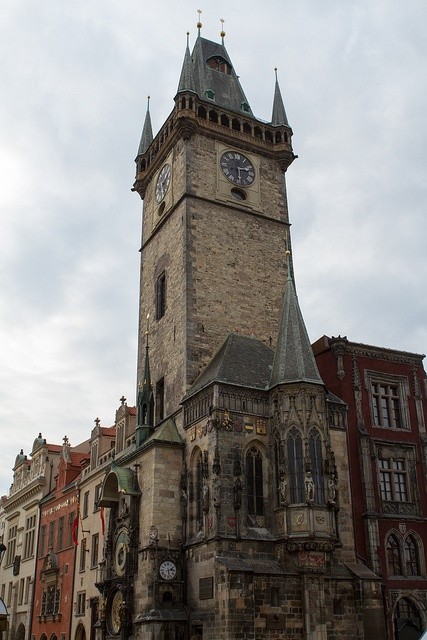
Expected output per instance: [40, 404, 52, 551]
[100, 506, 105, 536]
[71, 516, 79, 546]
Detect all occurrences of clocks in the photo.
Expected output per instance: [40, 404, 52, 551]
[153, 163, 172, 203]
[218, 149, 256, 188]
[159, 561, 177, 580]
